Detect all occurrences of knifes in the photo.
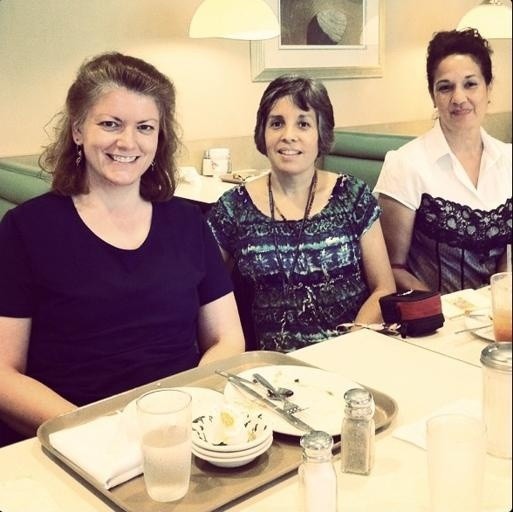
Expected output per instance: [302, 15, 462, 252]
[228, 379, 315, 433]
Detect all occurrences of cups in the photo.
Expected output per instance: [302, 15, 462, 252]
[136, 388, 191, 502]
[490, 272, 513, 342]
[211, 148, 228, 179]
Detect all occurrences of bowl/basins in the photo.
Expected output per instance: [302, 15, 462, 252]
[191, 408, 274, 470]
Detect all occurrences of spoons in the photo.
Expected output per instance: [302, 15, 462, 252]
[215, 369, 293, 399]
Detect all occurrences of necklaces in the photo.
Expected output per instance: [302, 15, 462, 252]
[265, 169, 322, 330]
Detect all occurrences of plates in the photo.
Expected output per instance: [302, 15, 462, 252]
[221, 364, 365, 436]
[466, 306, 513, 342]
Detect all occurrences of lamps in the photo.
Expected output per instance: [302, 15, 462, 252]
[187, 2, 281, 42]
[454, 0, 513, 40]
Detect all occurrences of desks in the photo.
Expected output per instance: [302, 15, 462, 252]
[172, 169, 269, 214]
[0, 281, 511, 512]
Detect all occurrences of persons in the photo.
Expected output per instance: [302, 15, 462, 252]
[0, 52, 254, 443]
[201, 72, 399, 357]
[369, 25, 512, 296]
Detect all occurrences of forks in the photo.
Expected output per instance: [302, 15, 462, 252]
[253, 372, 309, 414]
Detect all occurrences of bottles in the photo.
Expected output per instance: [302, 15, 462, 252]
[341, 389, 376, 475]
[479, 342, 512, 460]
[298, 431, 337, 512]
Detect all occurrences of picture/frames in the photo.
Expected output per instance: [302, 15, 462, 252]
[251, 0, 386, 80]
